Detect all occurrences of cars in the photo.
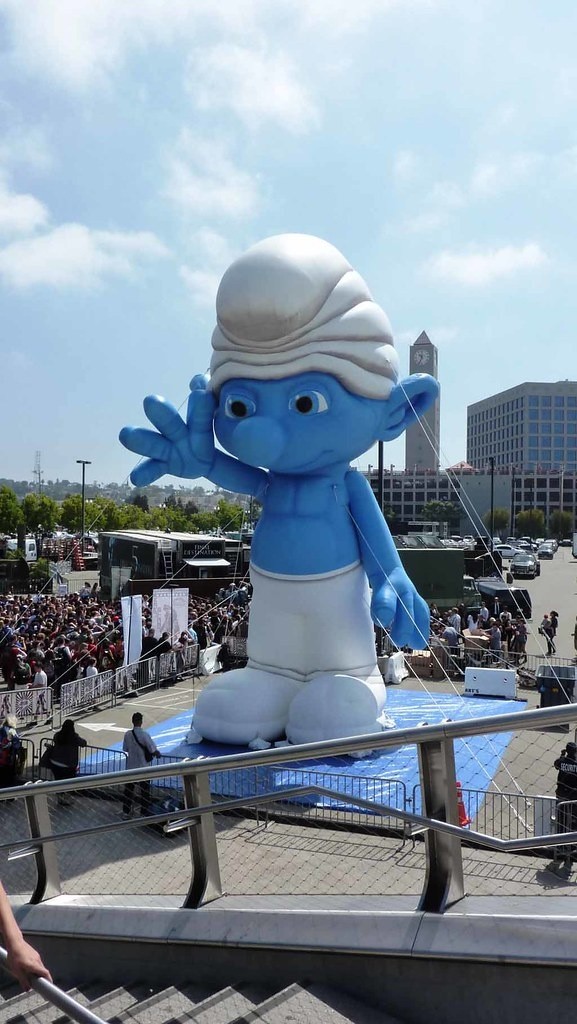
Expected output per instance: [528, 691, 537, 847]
[7, 526, 100, 551]
[427, 532, 577, 557]
[508, 553, 540, 578]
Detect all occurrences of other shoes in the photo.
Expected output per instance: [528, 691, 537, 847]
[121, 813, 147, 820]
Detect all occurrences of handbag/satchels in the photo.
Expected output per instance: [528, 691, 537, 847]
[141, 745, 154, 762]
[38, 745, 54, 769]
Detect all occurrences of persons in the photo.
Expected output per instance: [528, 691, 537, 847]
[553, 741, 576, 859]
[382, 596, 576, 664]
[53, 719, 87, 805]
[1, 883, 53, 992]
[0, 580, 251, 698]
[26, 661, 52, 726]
[122, 711, 161, 820]
[0, 713, 21, 803]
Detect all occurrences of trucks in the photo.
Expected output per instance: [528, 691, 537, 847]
[396, 547, 480, 624]
[474, 574, 532, 621]
